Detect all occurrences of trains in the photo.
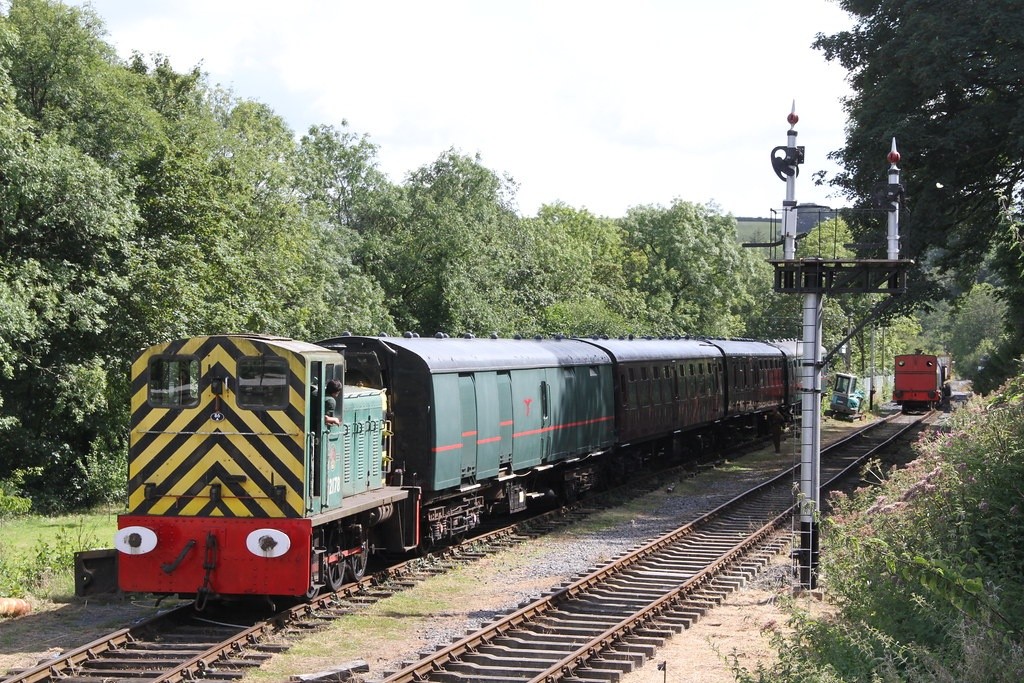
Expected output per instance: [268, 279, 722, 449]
[116, 333, 825, 606]
[893, 355, 952, 413]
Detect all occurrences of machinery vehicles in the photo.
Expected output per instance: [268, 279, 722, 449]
[829, 372, 868, 422]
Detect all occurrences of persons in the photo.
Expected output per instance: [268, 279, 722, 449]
[175, 369, 191, 404]
[939, 382, 952, 414]
[353, 378, 367, 387]
[310, 379, 343, 431]
